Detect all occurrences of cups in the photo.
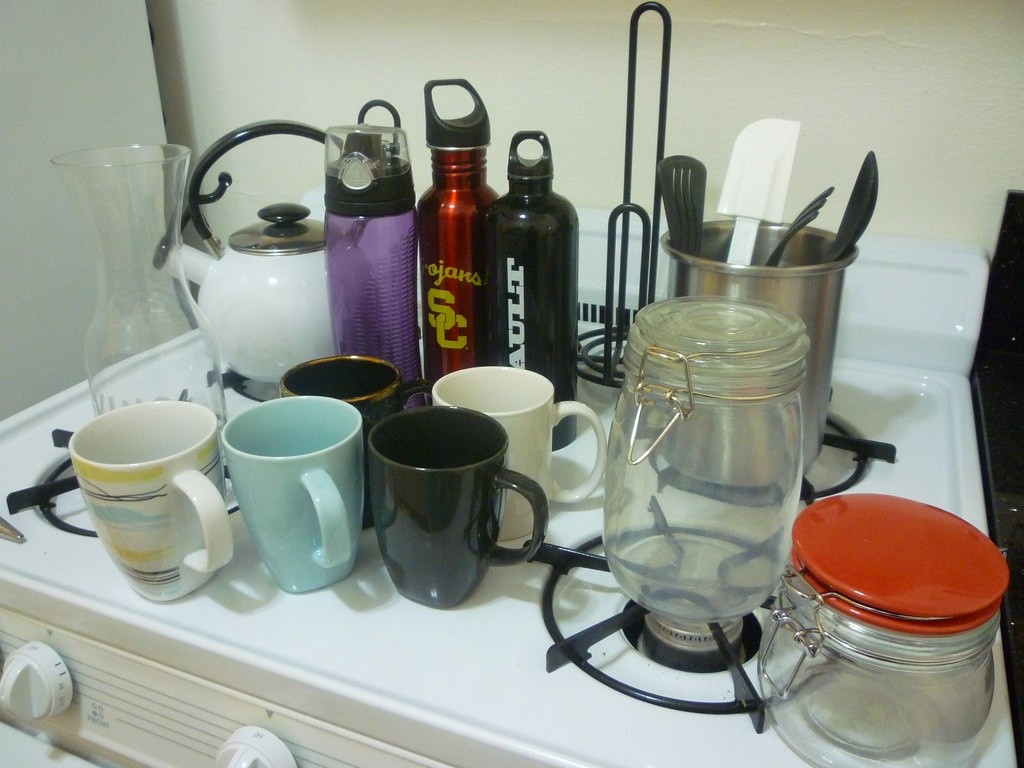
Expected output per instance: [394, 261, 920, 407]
[281, 355, 434, 530]
[69, 401, 234, 601]
[223, 397, 364, 592]
[432, 365, 607, 541]
[662, 222, 859, 486]
[366, 407, 549, 609]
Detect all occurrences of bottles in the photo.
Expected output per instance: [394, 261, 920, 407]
[324, 125, 422, 409]
[51, 144, 225, 460]
[757, 493, 1010, 768]
[605, 295, 811, 623]
[418, 79, 500, 406]
[484, 127, 579, 451]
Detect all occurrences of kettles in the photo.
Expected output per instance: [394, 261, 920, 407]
[154, 120, 344, 383]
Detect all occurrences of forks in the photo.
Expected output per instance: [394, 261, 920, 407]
[767, 186, 833, 267]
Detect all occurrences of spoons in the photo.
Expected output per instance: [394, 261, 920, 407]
[825, 152, 878, 262]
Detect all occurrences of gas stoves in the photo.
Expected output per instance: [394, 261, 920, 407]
[0, 209, 1020, 768]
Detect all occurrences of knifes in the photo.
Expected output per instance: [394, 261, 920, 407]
[718, 118, 800, 266]
